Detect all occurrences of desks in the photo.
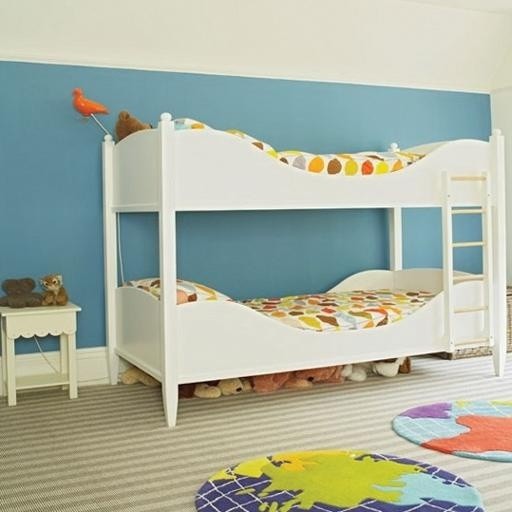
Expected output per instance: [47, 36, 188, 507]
[0, 301, 82, 407]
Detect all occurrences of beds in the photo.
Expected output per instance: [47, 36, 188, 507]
[102, 112, 508, 427]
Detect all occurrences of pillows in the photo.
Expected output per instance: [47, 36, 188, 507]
[129, 276, 234, 304]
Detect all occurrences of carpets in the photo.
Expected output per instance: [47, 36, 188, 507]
[393, 400, 512, 463]
[195, 450, 485, 512]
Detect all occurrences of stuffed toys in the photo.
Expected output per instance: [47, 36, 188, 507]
[121, 356, 410, 399]
[0, 278, 43, 308]
[41, 274, 68, 306]
[116, 111, 151, 141]
